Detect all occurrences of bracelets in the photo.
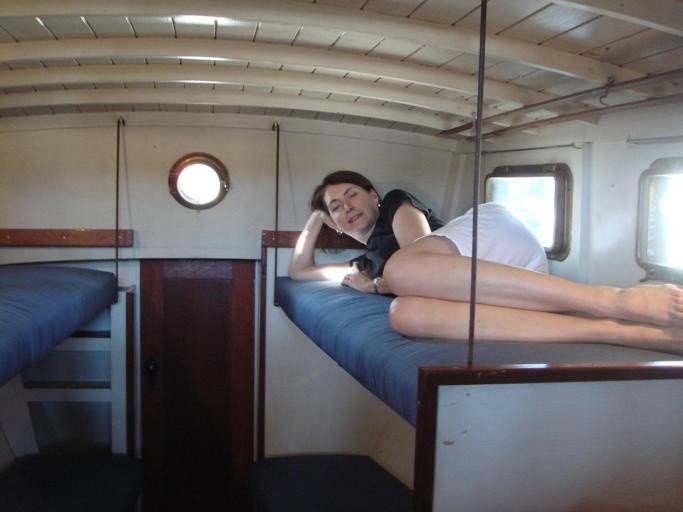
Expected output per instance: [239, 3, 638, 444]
[374, 277, 380, 294]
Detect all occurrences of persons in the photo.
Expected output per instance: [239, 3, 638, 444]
[288, 171, 683, 355]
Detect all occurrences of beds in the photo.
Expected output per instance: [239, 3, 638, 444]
[0, 229, 132, 511]
[256, 231, 682, 512]
